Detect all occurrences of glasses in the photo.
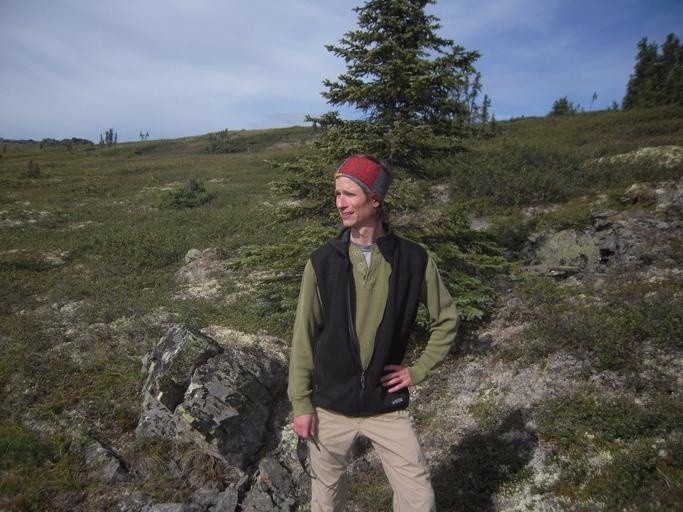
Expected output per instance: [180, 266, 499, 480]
[297, 432, 320, 480]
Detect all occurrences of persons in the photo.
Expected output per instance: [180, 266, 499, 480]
[287, 156, 460, 512]
